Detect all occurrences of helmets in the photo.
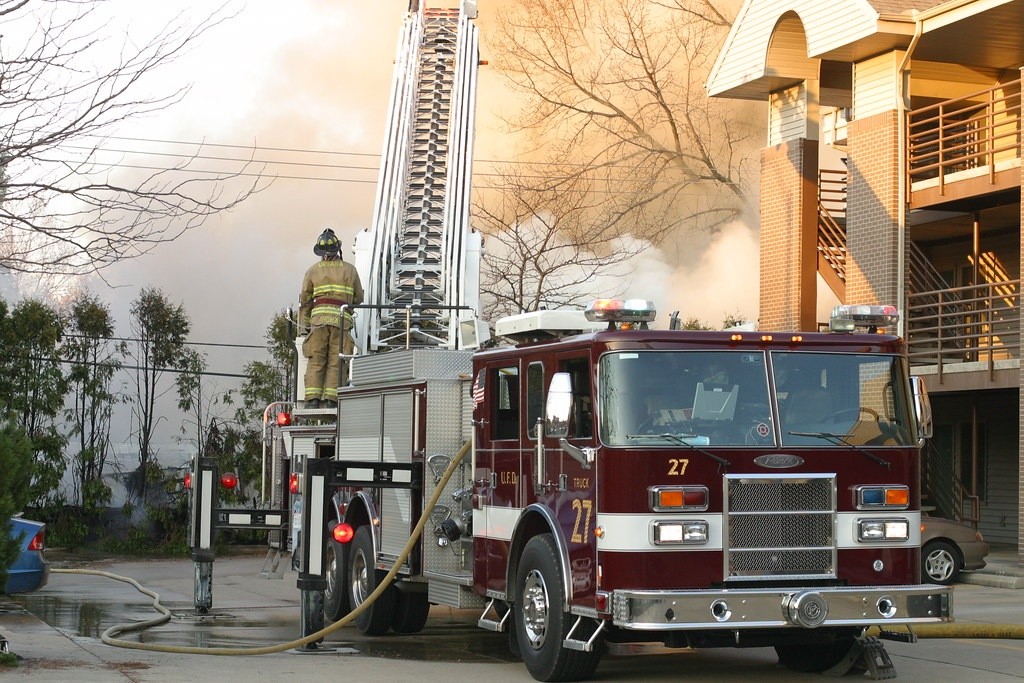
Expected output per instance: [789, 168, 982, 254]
[314, 229, 342, 256]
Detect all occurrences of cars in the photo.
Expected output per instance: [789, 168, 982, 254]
[919, 519, 989, 584]
[1, 516, 50, 596]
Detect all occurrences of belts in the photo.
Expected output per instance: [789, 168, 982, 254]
[316, 298, 346, 306]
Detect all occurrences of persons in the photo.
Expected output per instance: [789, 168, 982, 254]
[298, 228, 364, 409]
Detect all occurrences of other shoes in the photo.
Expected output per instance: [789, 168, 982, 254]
[304, 397, 320, 408]
[319, 399, 338, 408]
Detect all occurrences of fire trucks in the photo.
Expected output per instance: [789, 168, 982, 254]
[183, 0, 953, 683]
